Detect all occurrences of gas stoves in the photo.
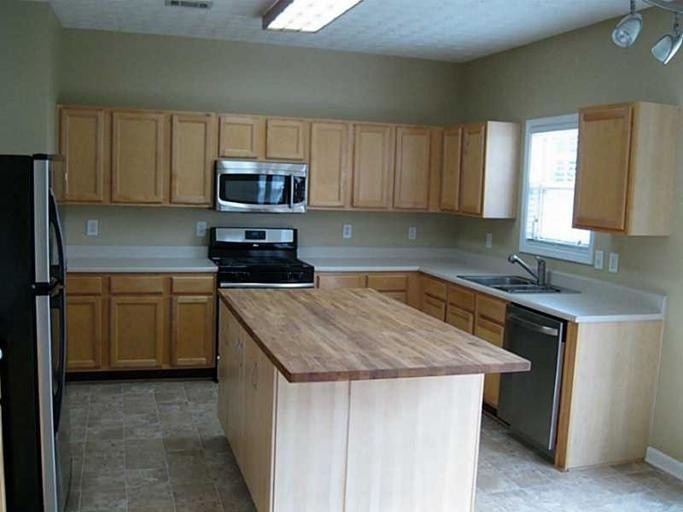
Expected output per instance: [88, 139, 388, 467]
[208, 226, 312, 269]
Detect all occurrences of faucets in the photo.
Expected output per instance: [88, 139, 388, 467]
[508, 253, 546, 286]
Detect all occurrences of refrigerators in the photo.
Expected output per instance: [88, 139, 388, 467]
[0, 150, 73, 511]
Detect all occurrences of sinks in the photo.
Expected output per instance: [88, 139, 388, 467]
[456, 274, 537, 286]
[492, 285, 580, 292]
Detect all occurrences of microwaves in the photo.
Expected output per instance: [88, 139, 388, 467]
[213, 160, 308, 215]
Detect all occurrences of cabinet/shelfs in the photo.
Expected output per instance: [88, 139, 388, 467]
[571, 101, 683, 239]
[316, 269, 419, 314]
[62, 270, 217, 380]
[419, 272, 508, 350]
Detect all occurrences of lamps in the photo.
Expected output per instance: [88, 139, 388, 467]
[609, 1, 682, 66]
